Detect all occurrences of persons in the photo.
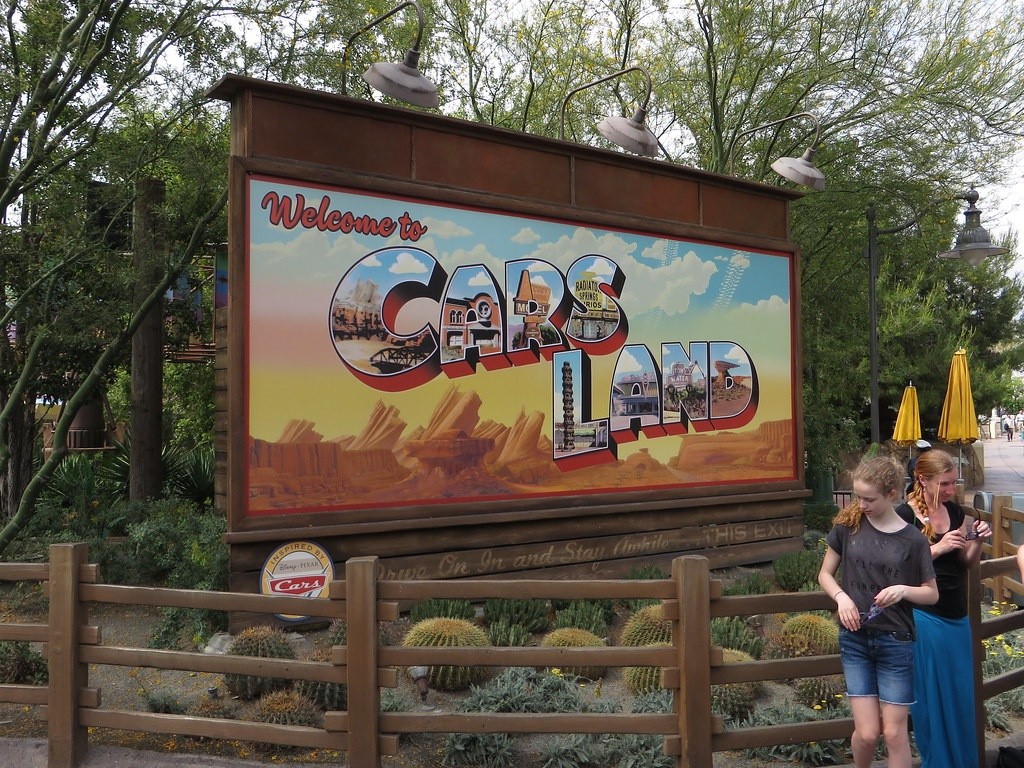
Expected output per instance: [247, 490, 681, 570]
[1016, 410, 1024, 436]
[907, 440, 932, 493]
[818, 455, 939, 768]
[895, 449, 992, 768]
[1004, 415, 1014, 442]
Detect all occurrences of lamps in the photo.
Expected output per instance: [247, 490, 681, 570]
[730, 111, 827, 191]
[561, 65, 659, 157]
[341, 1, 442, 110]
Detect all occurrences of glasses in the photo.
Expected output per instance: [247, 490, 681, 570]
[962, 507, 980, 541]
[860, 602, 884, 626]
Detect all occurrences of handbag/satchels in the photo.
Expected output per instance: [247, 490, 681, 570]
[1004, 423, 1009, 430]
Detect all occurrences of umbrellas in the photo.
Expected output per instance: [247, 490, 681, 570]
[937, 346, 979, 478]
[893, 386, 921, 458]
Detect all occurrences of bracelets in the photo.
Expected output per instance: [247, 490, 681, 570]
[834, 590, 843, 599]
